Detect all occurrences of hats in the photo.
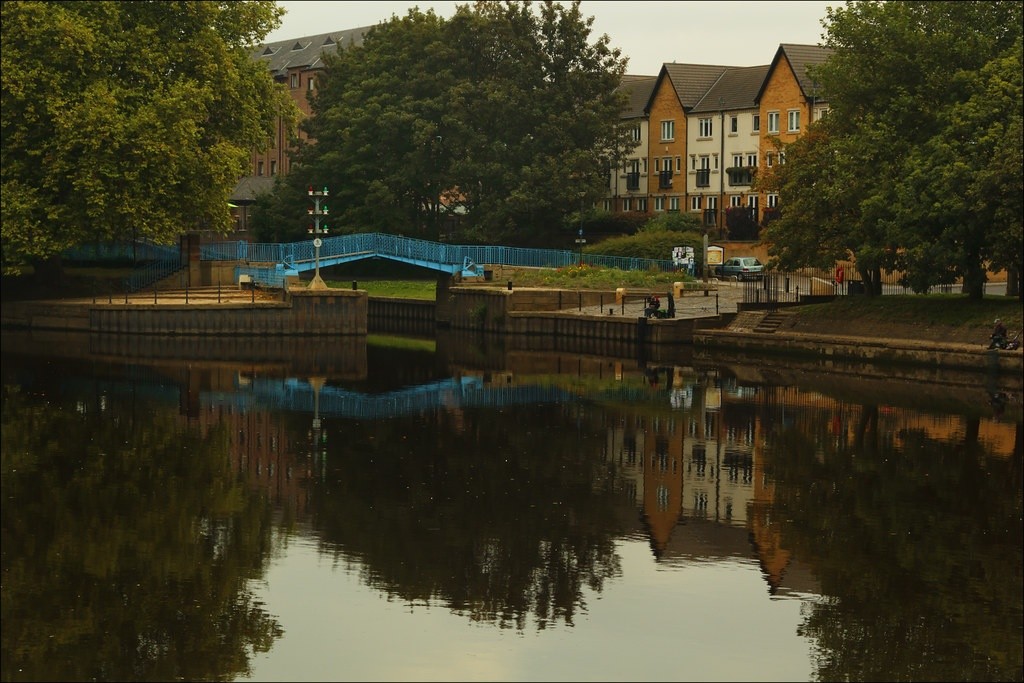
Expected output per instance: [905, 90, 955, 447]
[994, 319, 1001, 324]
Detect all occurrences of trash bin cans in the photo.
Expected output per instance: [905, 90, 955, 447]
[847, 279, 865, 296]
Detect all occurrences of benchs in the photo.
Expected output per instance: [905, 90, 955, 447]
[644, 308, 666, 318]
[674, 282, 718, 299]
[616, 287, 652, 306]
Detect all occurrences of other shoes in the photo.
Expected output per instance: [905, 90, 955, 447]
[987, 347, 995, 350]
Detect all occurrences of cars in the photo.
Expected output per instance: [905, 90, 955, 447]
[715, 257, 766, 280]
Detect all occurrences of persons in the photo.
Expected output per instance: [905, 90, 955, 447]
[989, 318, 1007, 349]
[645, 296, 660, 318]
